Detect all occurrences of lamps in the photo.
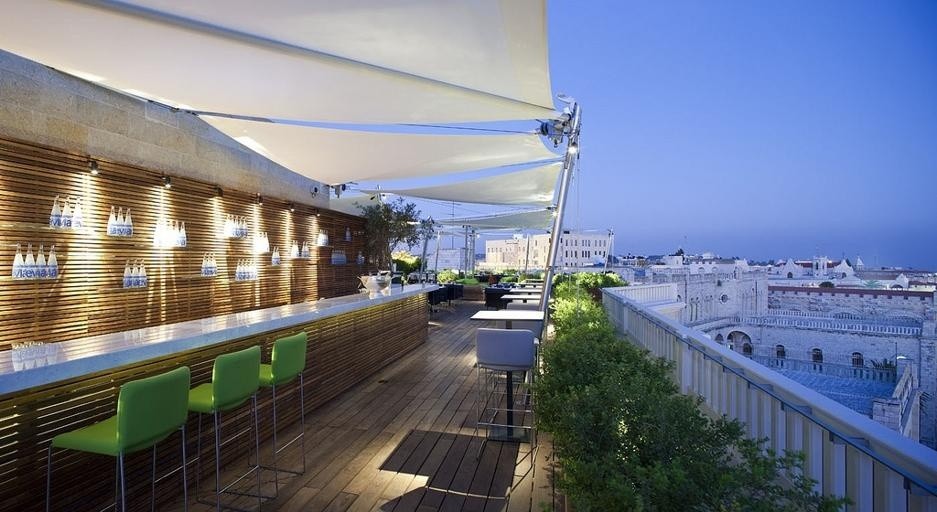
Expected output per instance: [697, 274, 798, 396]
[251, 193, 263, 205]
[208, 186, 223, 200]
[282, 202, 295, 213]
[159, 175, 171, 190]
[87, 160, 98, 176]
[309, 208, 320, 217]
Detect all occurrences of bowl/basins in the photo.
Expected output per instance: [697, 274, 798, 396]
[361, 275, 392, 291]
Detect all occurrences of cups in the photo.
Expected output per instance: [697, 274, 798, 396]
[11, 341, 43, 347]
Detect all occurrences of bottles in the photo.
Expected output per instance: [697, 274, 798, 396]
[236, 260, 258, 282]
[156, 219, 187, 248]
[198, 252, 218, 276]
[12, 343, 58, 376]
[49, 195, 84, 228]
[122, 258, 147, 288]
[225, 214, 248, 237]
[13, 243, 60, 279]
[422, 279, 456, 286]
[255, 227, 378, 266]
[106, 205, 133, 237]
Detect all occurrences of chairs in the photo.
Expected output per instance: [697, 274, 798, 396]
[221, 331, 307, 499]
[186, 346, 265, 511]
[45, 366, 191, 512]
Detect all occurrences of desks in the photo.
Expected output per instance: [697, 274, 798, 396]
[392, 271, 546, 465]
